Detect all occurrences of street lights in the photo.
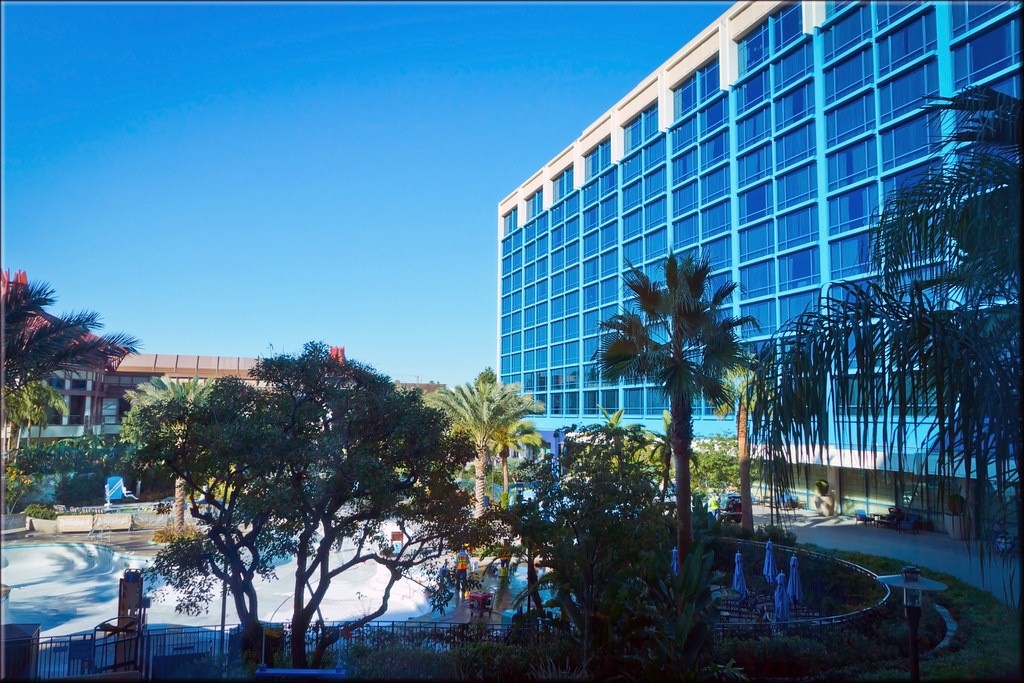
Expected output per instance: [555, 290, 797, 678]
[874, 565, 948, 683]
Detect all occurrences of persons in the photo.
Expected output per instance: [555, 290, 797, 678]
[500, 537, 511, 567]
[454, 546, 470, 592]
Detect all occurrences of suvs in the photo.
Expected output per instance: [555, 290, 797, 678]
[715, 495, 742, 522]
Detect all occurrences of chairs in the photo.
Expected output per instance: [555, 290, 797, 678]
[51, 500, 177, 513]
[749, 490, 933, 538]
[716, 589, 823, 638]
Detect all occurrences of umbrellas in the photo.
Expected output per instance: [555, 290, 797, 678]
[733, 550, 748, 593]
[787, 551, 802, 600]
[671, 546, 681, 575]
[773, 567, 790, 620]
[763, 537, 779, 580]
